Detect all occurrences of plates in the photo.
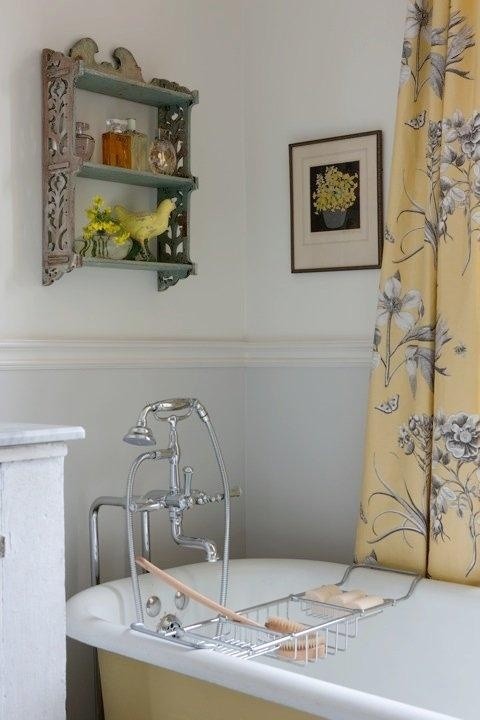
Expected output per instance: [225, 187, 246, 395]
[0, 455, 66, 720]
[49, 40, 190, 288]
[76, 167, 188, 264]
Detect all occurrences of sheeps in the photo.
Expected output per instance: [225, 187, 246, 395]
[168, 463, 218, 562]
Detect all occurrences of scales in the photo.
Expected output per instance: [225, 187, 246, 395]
[65, 558, 478, 716]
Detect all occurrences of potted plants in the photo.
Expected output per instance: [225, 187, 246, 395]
[288, 130, 384, 274]
